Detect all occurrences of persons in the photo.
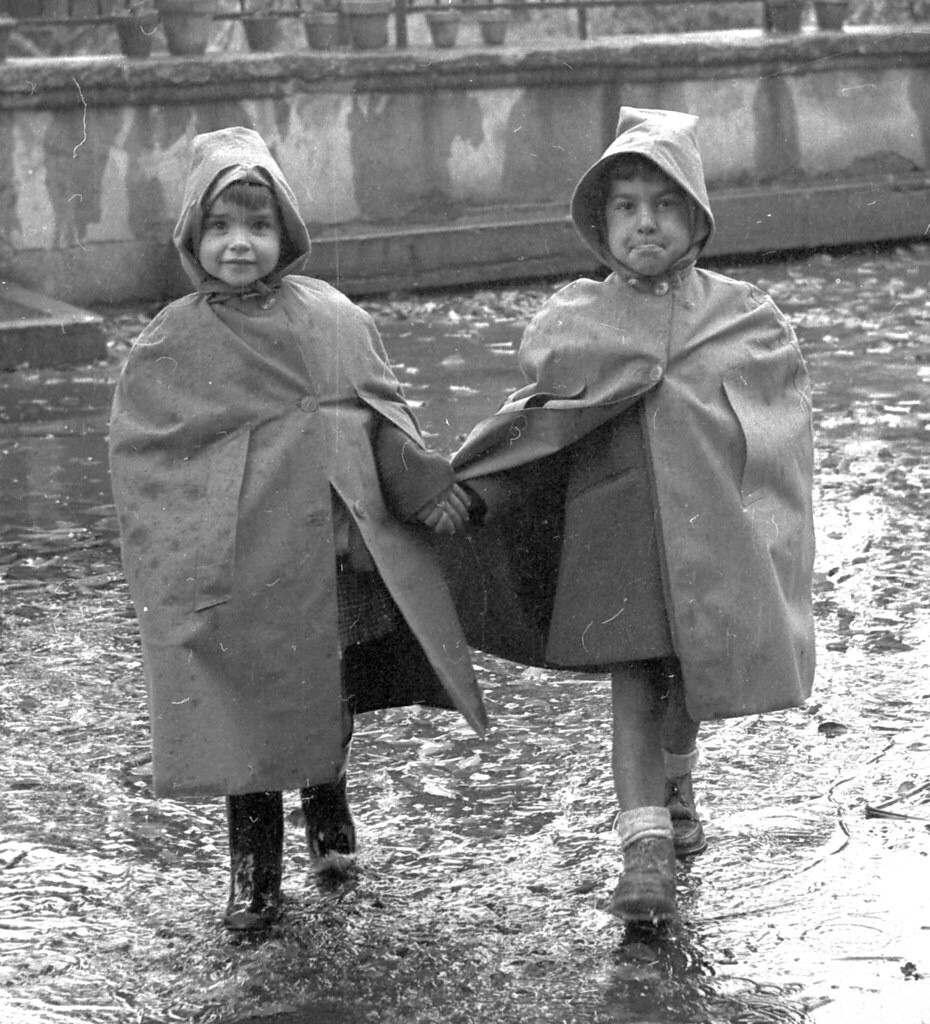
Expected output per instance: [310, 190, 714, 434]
[111, 126, 471, 935]
[418, 108, 817, 921]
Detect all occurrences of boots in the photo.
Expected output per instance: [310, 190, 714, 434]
[226, 791, 283, 930]
[297, 767, 356, 898]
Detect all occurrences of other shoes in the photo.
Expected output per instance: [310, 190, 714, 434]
[616, 806, 678, 927]
[660, 747, 708, 857]
